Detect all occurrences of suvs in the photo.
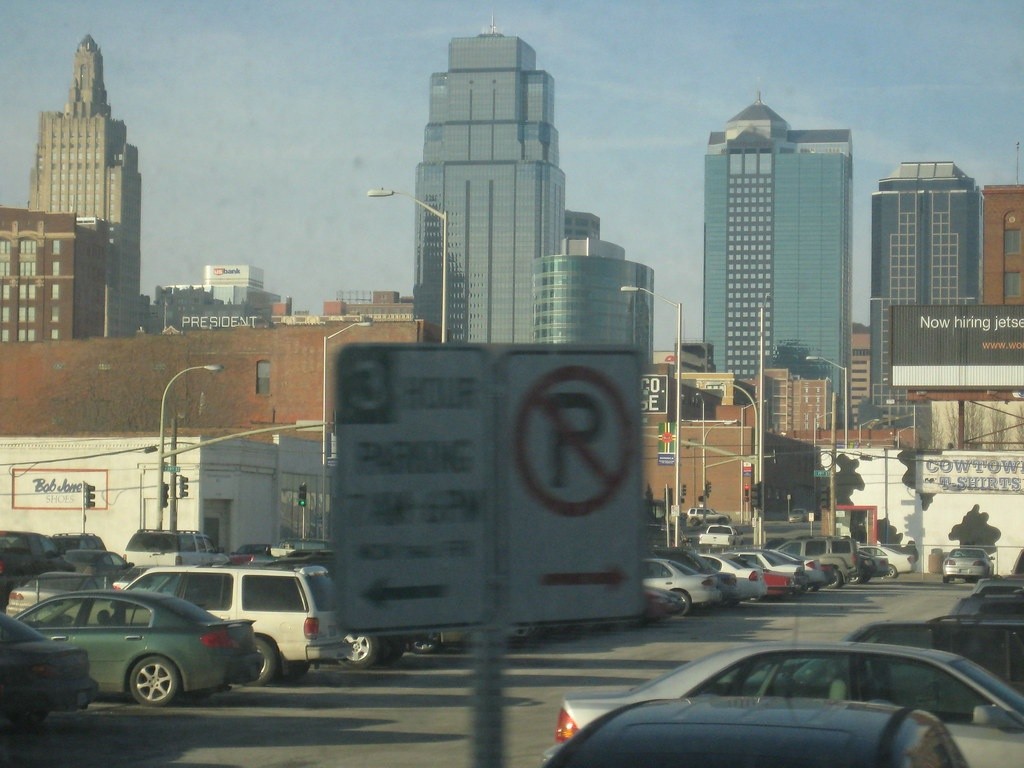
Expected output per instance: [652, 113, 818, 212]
[120, 564, 353, 688]
[687, 508, 732, 526]
[776, 538, 860, 589]
[124, 529, 229, 566]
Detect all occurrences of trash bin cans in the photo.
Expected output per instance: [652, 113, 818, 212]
[928, 548, 943, 574]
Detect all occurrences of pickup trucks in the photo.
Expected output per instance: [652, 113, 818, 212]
[698, 525, 744, 545]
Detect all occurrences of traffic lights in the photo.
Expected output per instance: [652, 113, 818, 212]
[86, 486, 95, 509]
[745, 484, 749, 496]
[162, 482, 169, 508]
[682, 485, 686, 496]
[180, 476, 188, 499]
[751, 483, 762, 507]
[298, 483, 307, 506]
[706, 484, 711, 497]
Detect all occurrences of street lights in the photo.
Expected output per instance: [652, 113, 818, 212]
[368, 190, 448, 343]
[695, 393, 732, 524]
[859, 419, 879, 443]
[806, 356, 848, 449]
[157, 365, 224, 530]
[741, 400, 768, 525]
[621, 286, 681, 546]
[897, 425, 914, 449]
[814, 411, 833, 511]
[322, 321, 374, 542]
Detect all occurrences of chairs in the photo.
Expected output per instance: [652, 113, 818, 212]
[97, 609, 110, 626]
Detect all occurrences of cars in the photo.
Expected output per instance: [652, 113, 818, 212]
[788, 508, 809, 524]
[1, 529, 335, 607]
[0, 611, 101, 730]
[943, 548, 995, 584]
[644, 545, 916, 618]
[11, 590, 265, 708]
[543, 576, 1024, 768]
